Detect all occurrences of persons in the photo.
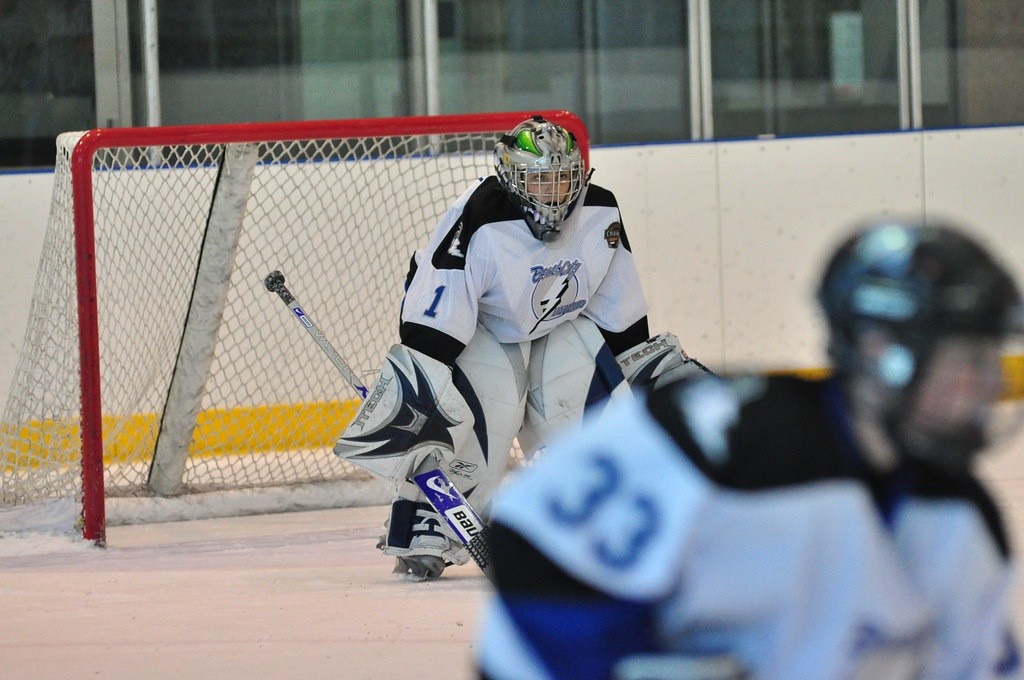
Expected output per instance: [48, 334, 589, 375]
[481, 222, 1024, 680]
[334, 114, 720, 582]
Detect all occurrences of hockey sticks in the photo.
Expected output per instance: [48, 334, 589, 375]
[263, 269, 490, 587]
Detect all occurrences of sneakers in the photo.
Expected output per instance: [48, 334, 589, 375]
[393, 556, 445, 582]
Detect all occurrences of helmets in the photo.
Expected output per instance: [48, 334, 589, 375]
[819, 224, 1024, 394]
[492, 115, 585, 242]
